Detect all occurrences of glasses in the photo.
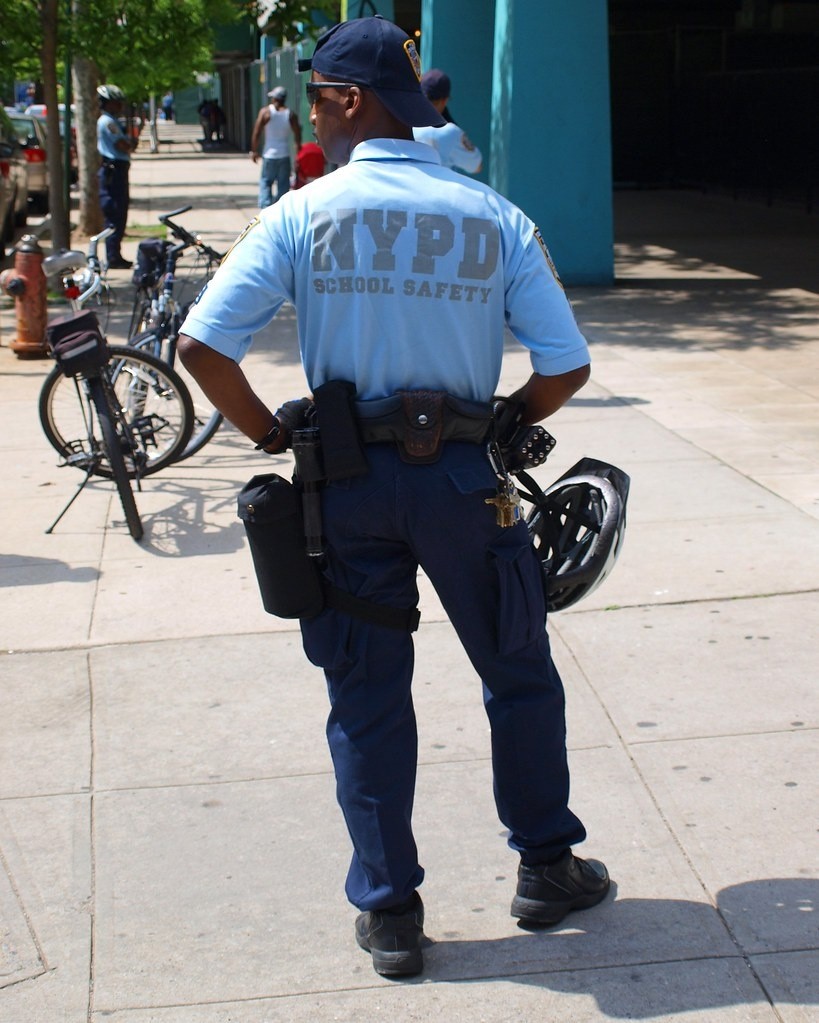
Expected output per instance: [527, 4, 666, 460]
[305, 81, 369, 109]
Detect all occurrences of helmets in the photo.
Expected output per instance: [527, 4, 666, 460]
[98, 85, 125, 101]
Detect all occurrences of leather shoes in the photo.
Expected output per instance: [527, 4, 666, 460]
[354, 893, 425, 977]
[510, 848, 610, 924]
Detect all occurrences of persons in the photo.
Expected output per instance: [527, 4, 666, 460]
[249, 86, 302, 209]
[96, 84, 133, 268]
[198, 99, 222, 143]
[176, 13, 611, 976]
[412, 69, 483, 174]
[162, 92, 173, 121]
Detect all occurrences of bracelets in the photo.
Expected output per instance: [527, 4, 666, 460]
[254, 417, 281, 451]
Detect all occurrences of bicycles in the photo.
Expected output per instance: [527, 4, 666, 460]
[120, 205, 232, 467]
[38, 221, 197, 543]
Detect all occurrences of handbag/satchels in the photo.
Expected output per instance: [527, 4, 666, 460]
[237, 474, 325, 620]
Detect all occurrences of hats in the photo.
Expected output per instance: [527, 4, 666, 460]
[419, 70, 450, 100]
[297, 14, 446, 127]
[268, 86, 287, 99]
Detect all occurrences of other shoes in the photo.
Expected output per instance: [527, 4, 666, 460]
[108, 259, 132, 269]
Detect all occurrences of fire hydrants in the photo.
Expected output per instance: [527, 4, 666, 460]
[0, 234, 60, 362]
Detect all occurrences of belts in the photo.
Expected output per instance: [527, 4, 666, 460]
[307, 389, 495, 446]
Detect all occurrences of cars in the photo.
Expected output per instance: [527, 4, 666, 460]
[0, 104, 37, 259]
[25, 102, 79, 186]
[5, 108, 52, 214]
